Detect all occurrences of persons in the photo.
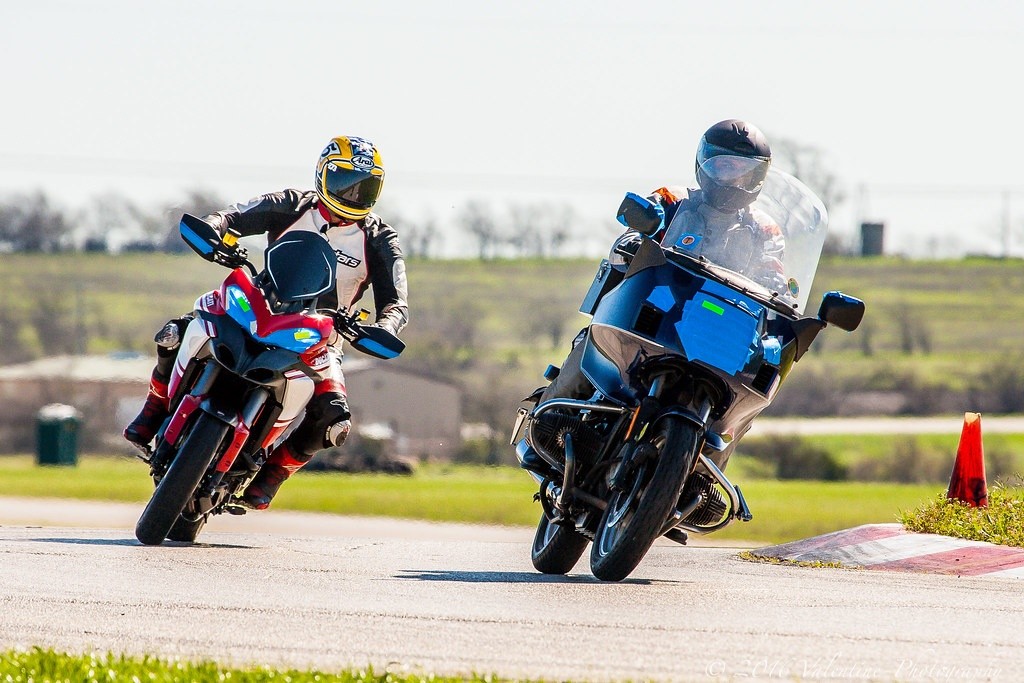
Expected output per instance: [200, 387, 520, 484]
[522, 117, 790, 488]
[124, 137, 410, 512]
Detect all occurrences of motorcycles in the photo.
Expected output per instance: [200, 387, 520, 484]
[132, 213, 408, 547]
[509, 190, 866, 584]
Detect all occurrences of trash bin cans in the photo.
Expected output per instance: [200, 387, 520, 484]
[34, 403, 80, 467]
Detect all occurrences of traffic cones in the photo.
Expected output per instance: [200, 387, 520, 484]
[945, 410, 987, 509]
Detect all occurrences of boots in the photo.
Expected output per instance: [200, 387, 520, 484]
[242, 447, 315, 509]
[124, 368, 174, 445]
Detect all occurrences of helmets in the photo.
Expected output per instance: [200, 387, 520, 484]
[695, 120, 772, 210]
[315, 135, 385, 220]
